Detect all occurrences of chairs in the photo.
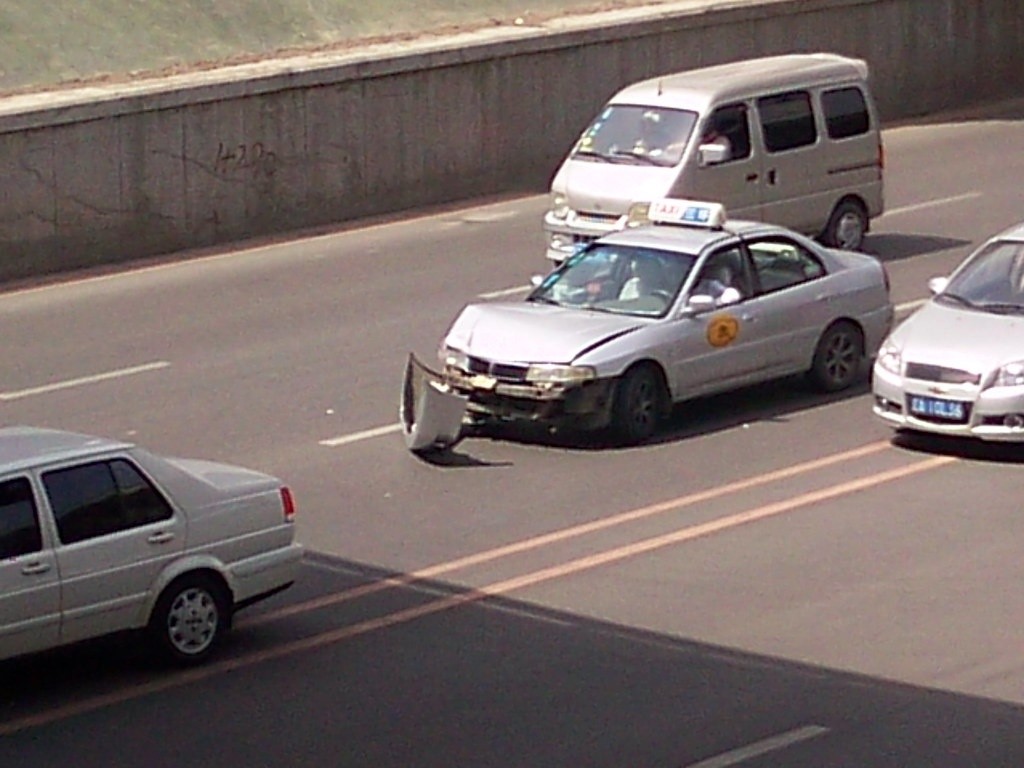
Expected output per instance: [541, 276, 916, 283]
[759, 268, 796, 295]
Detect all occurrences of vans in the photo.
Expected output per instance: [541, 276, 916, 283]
[545, 54, 885, 281]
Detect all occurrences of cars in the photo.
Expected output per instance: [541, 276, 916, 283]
[0, 422, 309, 675]
[872, 220, 1023, 444]
[437, 195, 894, 448]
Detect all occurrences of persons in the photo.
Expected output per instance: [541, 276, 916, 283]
[586, 258, 729, 305]
[608, 117, 730, 162]
